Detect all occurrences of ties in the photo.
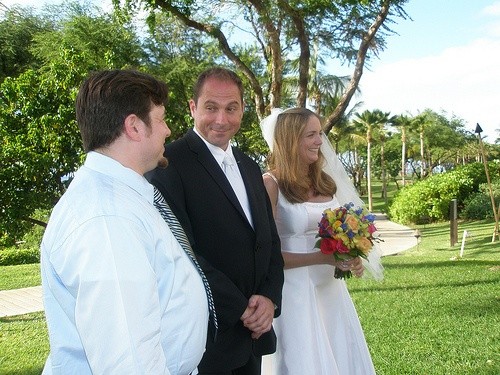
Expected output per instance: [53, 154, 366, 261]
[154, 186, 219, 329]
[223, 155, 252, 226]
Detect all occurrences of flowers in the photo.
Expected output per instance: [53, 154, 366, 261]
[313, 202, 385, 281]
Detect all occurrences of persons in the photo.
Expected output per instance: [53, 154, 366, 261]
[39, 69, 218, 375]
[144, 67, 285, 375]
[259, 107, 385, 375]
